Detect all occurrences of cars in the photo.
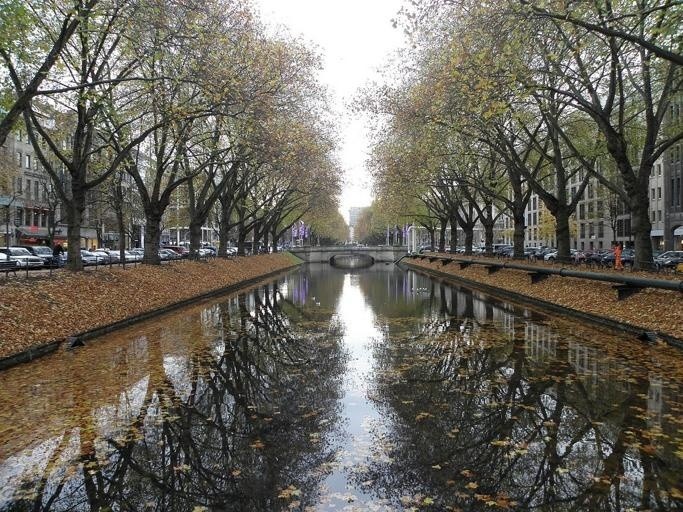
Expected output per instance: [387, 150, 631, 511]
[0, 246, 144, 271]
[227, 247, 239, 256]
[524, 247, 634, 267]
[653, 251, 683, 272]
[419, 245, 514, 257]
[159, 246, 216, 260]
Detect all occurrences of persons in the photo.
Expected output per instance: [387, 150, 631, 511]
[52, 243, 64, 267]
[612, 241, 625, 271]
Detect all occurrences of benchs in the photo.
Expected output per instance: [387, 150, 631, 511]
[0, 261, 21, 276]
[426, 247, 683, 276]
